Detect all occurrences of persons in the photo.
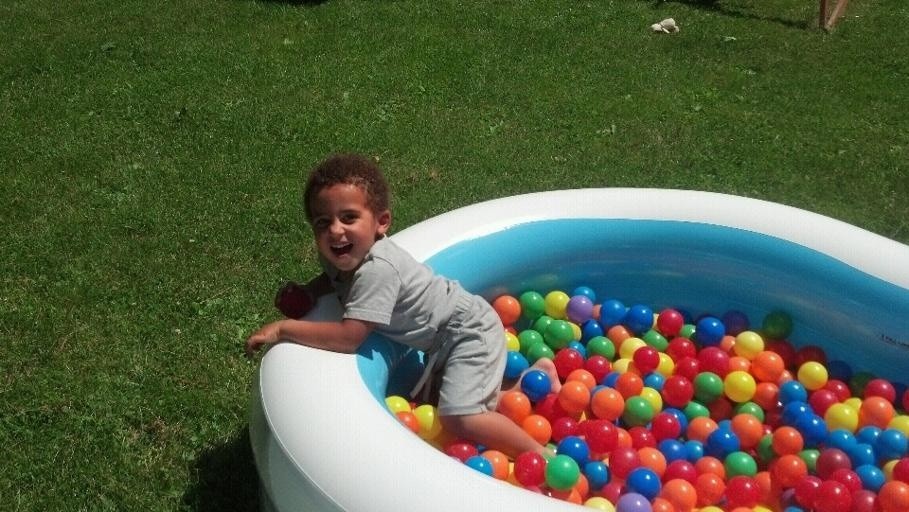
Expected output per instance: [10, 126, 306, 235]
[244, 155, 554, 463]
